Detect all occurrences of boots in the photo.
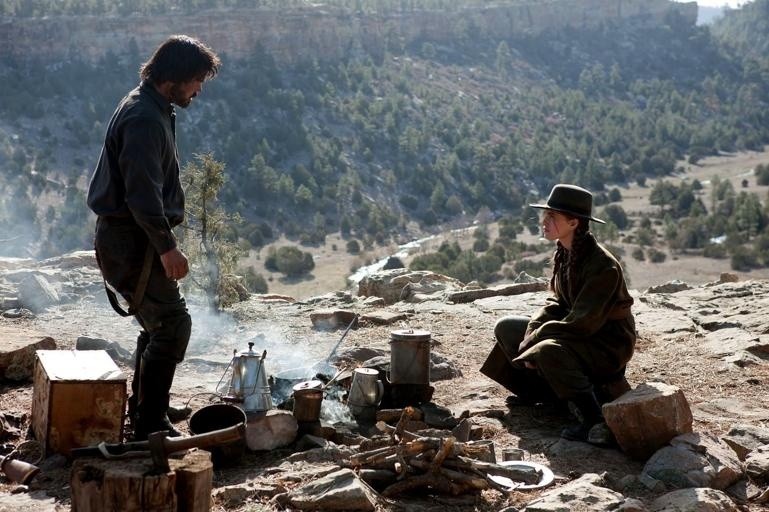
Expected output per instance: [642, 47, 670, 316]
[128, 337, 192, 441]
[507, 395, 535, 405]
[560, 390, 605, 441]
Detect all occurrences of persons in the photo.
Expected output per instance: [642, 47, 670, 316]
[479, 185, 638, 444]
[87, 35, 220, 455]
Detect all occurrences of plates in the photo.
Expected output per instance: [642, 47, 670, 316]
[488, 461, 555, 489]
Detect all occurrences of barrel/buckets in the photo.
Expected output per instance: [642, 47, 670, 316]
[185, 393, 247, 462]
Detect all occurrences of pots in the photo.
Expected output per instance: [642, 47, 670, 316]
[276, 365, 337, 391]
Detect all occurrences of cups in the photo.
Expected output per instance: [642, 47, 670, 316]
[470, 439, 525, 462]
[293, 382, 322, 423]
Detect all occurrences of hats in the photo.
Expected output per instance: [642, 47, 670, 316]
[530, 184, 606, 224]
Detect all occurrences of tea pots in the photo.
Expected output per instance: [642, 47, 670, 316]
[216, 342, 273, 413]
[347, 367, 384, 422]
